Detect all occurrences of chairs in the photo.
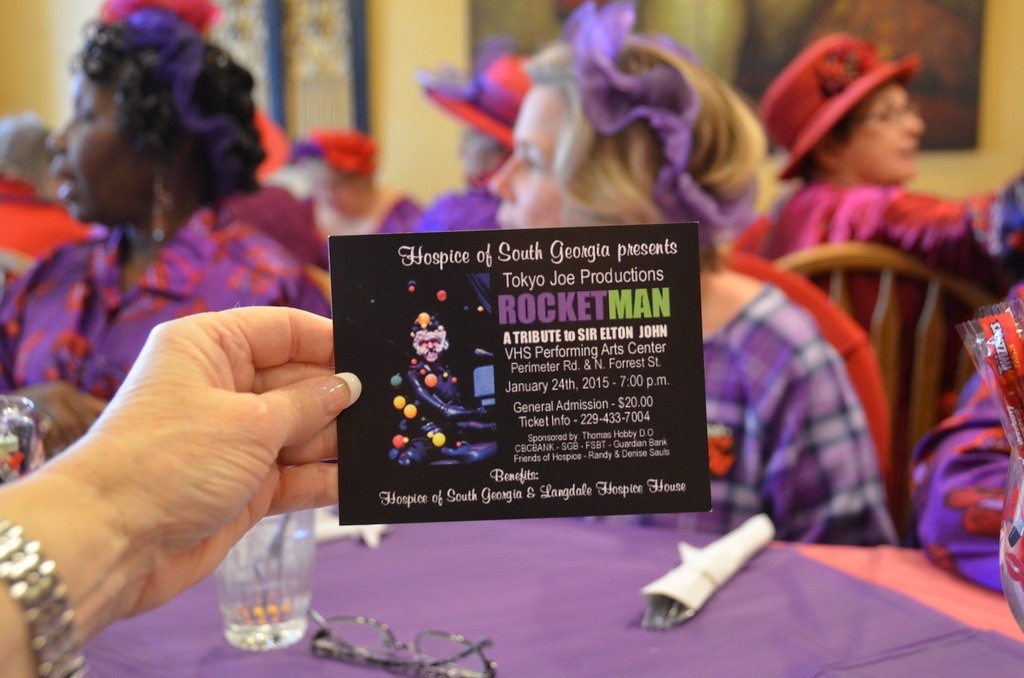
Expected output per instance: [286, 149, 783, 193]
[771, 241, 998, 549]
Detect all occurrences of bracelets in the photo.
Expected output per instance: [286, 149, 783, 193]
[0, 519, 94, 678]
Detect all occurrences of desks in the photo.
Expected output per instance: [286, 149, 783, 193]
[76, 518, 1024, 678]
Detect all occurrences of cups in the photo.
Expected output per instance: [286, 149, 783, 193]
[215, 508, 316, 652]
[0, 395, 45, 485]
[999, 450, 1024, 634]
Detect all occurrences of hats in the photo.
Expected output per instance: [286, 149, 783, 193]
[411, 32, 534, 153]
[759, 32, 927, 184]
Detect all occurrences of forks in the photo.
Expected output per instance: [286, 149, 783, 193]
[641, 597, 681, 635]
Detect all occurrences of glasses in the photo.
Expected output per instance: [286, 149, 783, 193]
[303, 603, 497, 678]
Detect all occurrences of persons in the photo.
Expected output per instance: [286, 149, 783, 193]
[0, 304, 360, 678]
[1, 0, 1024, 592]
